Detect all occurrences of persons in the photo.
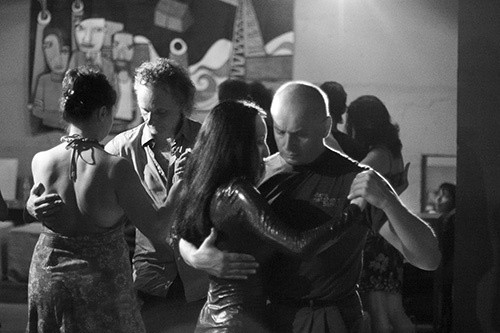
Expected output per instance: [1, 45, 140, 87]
[170, 100, 368, 333]
[26, 60, 456, 333]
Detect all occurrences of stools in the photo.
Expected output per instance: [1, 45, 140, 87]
[6, 222, 45, 286]
[0, 220, 14, 280]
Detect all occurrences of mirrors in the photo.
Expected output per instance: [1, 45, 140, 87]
[418, 151, 456, 217]
